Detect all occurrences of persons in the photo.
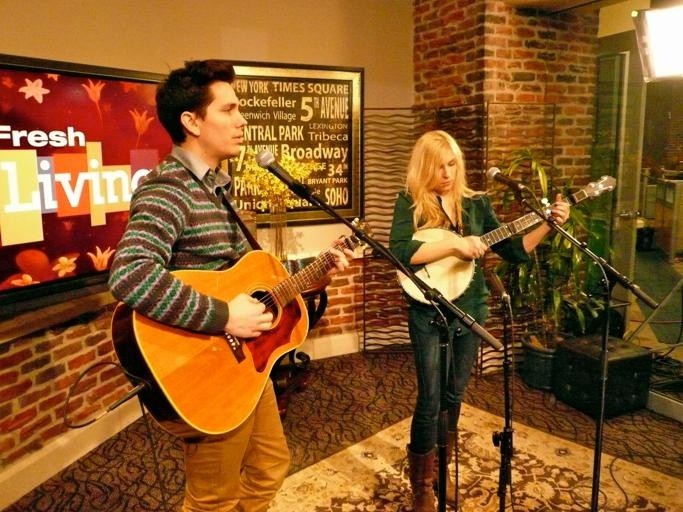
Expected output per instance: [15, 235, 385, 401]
[382, 127, 574, 510]
[104, 57, 356, 511]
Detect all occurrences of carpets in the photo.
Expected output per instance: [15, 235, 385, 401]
[262, 402, 683, 512]
[634, 249, 682, 346]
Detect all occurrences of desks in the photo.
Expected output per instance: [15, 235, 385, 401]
[562, 293, 631, 336]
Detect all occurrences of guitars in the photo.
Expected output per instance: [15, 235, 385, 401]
[113, 218, 375, 442]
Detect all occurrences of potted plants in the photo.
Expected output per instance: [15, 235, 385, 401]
[495, 151, 614, 391]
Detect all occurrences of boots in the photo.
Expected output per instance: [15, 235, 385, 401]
[406, 442, 436, 512]
[435, 424, 466, 510]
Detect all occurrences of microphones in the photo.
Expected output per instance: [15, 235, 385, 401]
[485, 167, 535, 197]
[255, 149, 321, 207]
